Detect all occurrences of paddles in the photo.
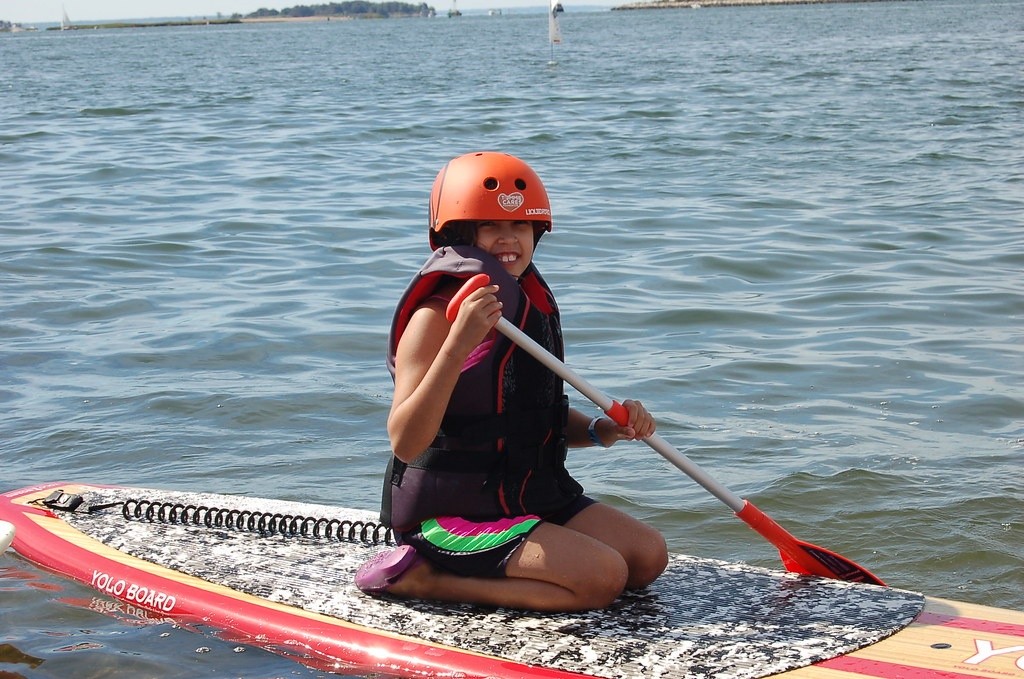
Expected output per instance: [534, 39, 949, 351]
[443, 273, 888, 585]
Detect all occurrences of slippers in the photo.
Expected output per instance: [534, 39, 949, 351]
[352, 545, 420, 596]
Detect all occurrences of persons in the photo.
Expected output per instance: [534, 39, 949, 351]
[353, 150, 668, 612]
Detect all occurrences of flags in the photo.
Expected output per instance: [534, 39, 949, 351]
[550, 0, 566, 45]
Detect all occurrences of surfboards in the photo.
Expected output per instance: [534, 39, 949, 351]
[0, 480, 1024, 678]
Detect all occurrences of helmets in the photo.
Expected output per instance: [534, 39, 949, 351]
[426, 150, 553, 252]
